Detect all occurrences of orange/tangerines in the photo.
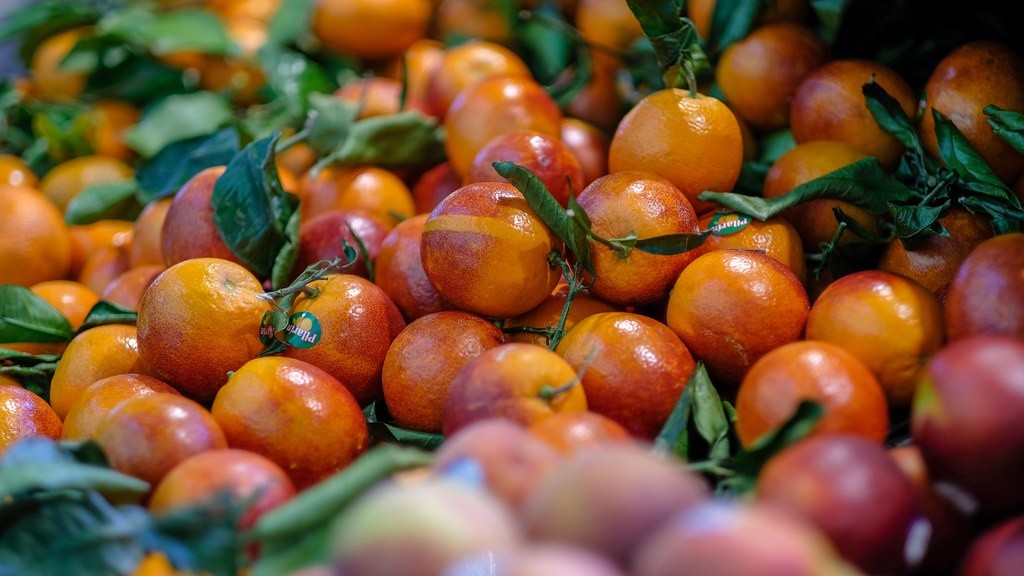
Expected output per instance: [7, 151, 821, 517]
[0, 0, 1024, 576]
